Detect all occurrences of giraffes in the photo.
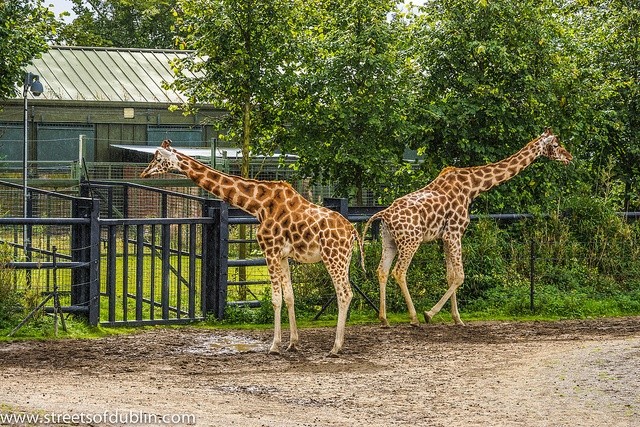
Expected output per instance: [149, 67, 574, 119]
[363, 126, 573, 328]
[140, 139, 367, 357]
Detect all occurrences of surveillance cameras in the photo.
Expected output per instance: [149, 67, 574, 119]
[30, 81, 44, 97]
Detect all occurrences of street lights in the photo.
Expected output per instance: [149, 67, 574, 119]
[22, 71, 44, 290]
